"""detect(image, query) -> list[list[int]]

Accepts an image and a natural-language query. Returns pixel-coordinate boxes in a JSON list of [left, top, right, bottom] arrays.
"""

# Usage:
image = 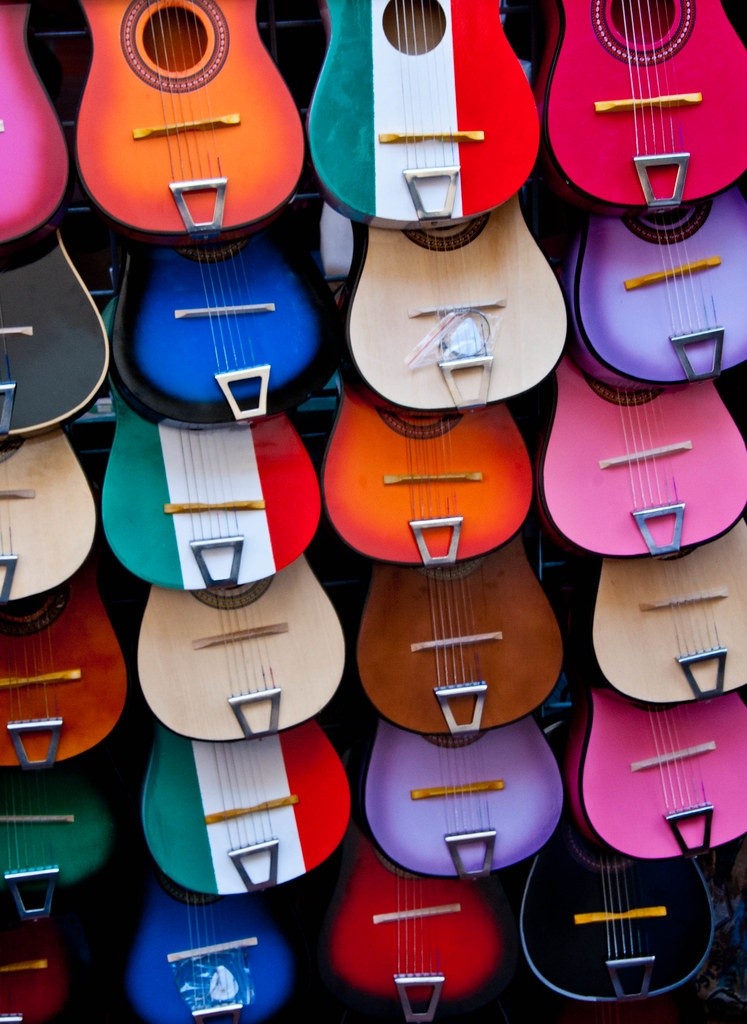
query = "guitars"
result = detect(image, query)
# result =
[[0, 2, 746, 1017]]
